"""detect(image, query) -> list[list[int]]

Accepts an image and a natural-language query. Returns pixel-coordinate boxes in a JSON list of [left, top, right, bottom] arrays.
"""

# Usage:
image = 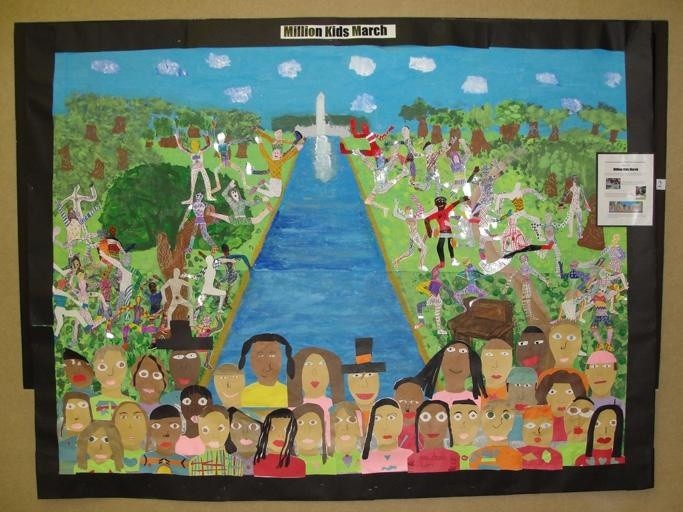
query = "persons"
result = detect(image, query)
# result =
[[51, 113, 627, 478]]
